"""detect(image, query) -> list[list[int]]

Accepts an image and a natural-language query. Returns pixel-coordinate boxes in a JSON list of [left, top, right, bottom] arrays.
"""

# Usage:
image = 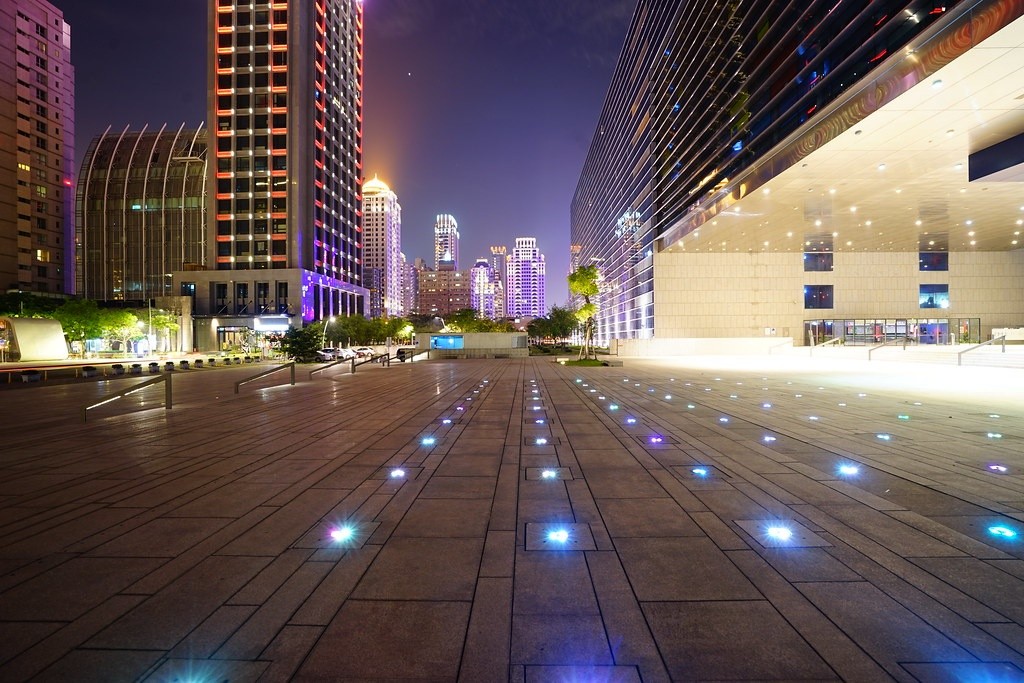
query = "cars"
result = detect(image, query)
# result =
[[294, 350, 332, 363], [354, 347, 375, 357], [322, 347, 365, 359]]
